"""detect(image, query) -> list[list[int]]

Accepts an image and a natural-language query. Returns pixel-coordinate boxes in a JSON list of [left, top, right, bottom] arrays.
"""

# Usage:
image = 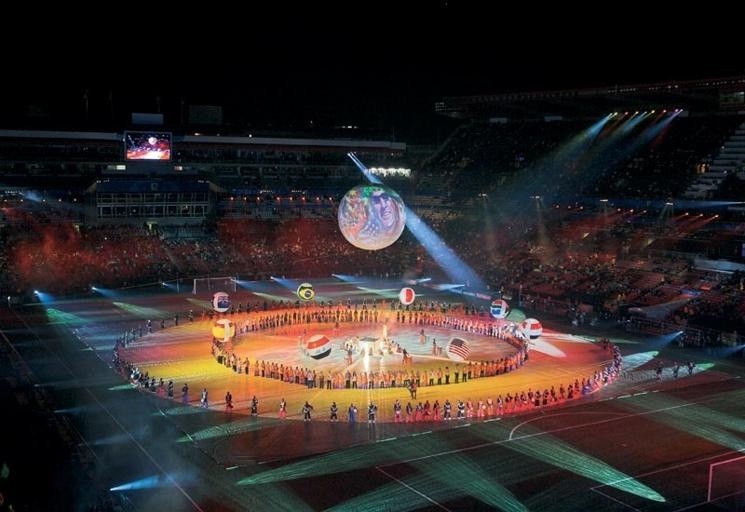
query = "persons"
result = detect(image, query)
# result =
[[409, 119, 744, 350], [671, 361, 681, 380], [1, 135, 408, 291], [653, 359, 666, 385], [686, 358, 694, 376], [110, 298, 621, 426]]
